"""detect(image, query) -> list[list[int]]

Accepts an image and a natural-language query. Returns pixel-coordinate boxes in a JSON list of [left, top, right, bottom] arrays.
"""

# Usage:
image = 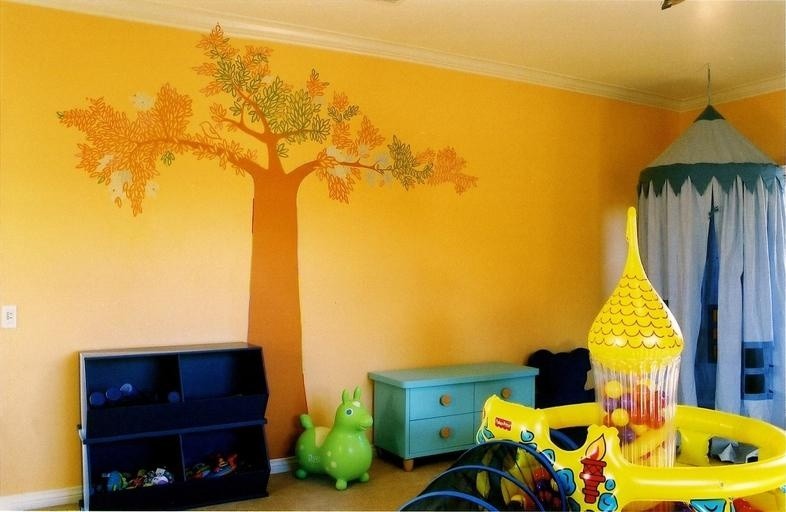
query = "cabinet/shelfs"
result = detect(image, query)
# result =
[[367, 361, 540, 472], [75, 342, 270, 510]]
